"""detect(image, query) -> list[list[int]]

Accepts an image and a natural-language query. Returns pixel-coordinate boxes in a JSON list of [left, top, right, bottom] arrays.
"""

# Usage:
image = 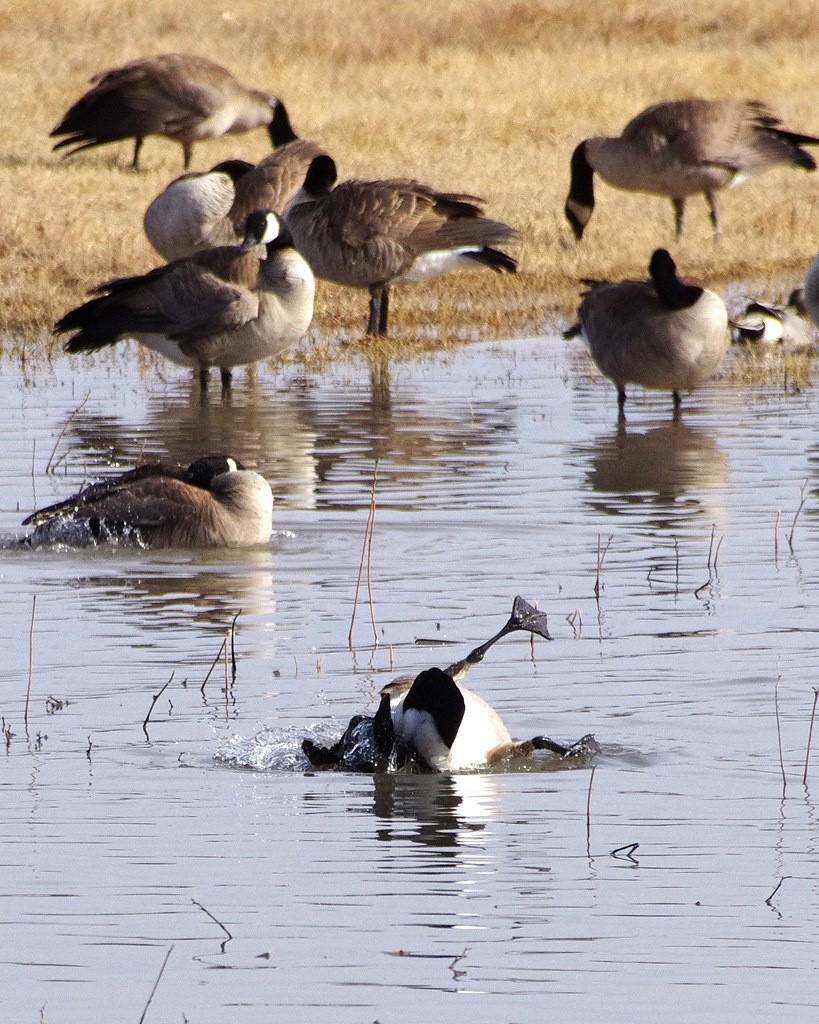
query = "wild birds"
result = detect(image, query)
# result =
[[52, 208, 316, 392], [282, 156, 521, 338], [144, 139, 326, 265], [47, 54, 302, 175], [17, 455, 273, 552], [726, 288, 816, 349], [561, 247, 732, 412], [563, 98, 819, 245], [302, 593, 604, 773]]
